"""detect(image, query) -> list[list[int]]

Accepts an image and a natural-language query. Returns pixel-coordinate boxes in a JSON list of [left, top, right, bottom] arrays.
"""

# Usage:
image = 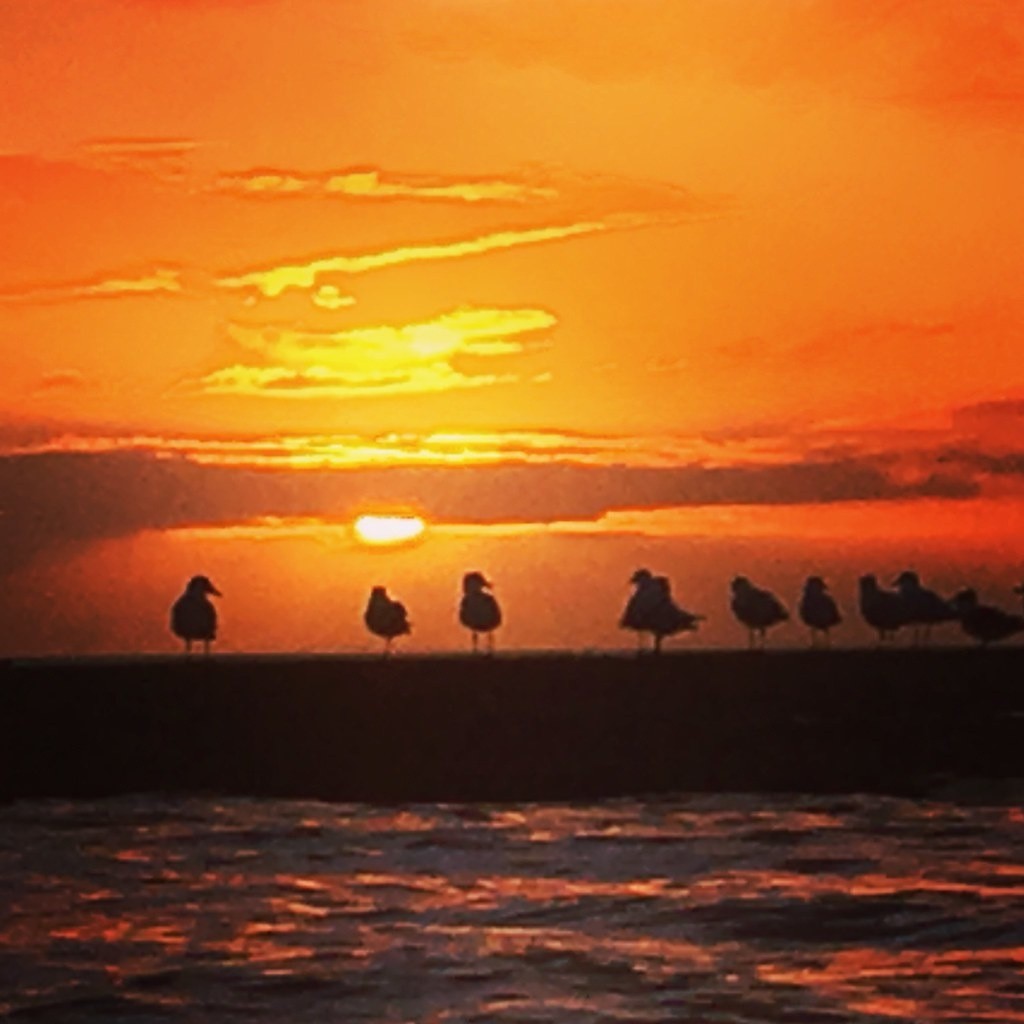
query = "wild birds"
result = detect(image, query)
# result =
[[362, 584, 412, 660], [857, 570, 1024, 650], [621, 568, 707, 661], [169, 574, 224, 664], [729, 574, 790, 657], [458, 570, 502, 662], [799, 575, 843, 653]]
